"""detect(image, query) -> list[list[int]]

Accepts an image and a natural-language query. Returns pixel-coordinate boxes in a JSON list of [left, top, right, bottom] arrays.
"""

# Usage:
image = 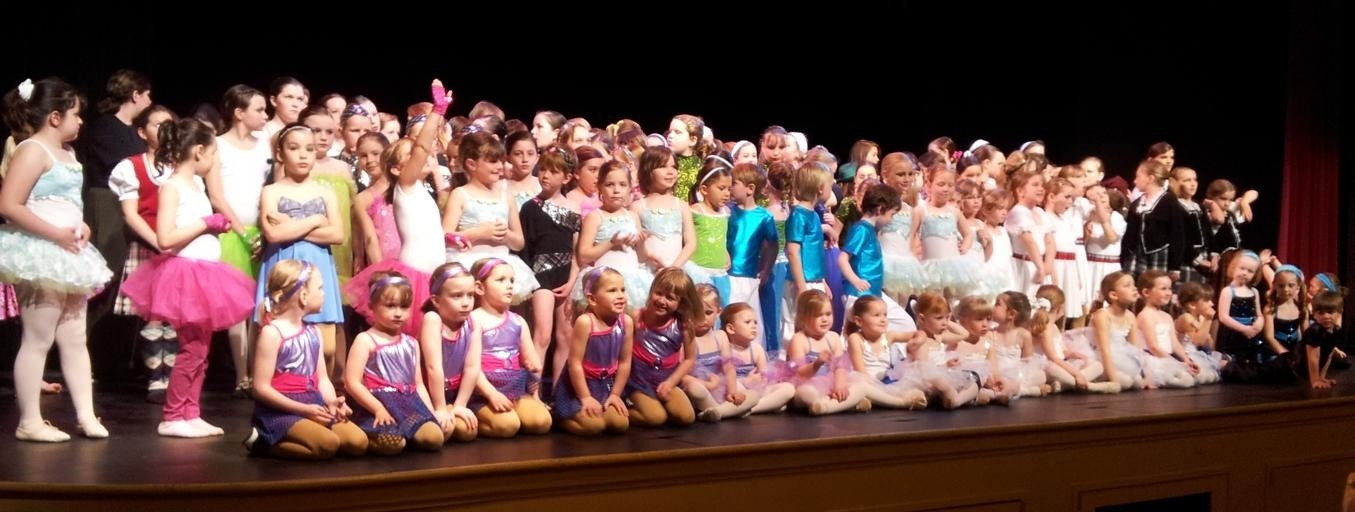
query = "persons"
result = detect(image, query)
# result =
[[0, 63, 1354, 444]]
